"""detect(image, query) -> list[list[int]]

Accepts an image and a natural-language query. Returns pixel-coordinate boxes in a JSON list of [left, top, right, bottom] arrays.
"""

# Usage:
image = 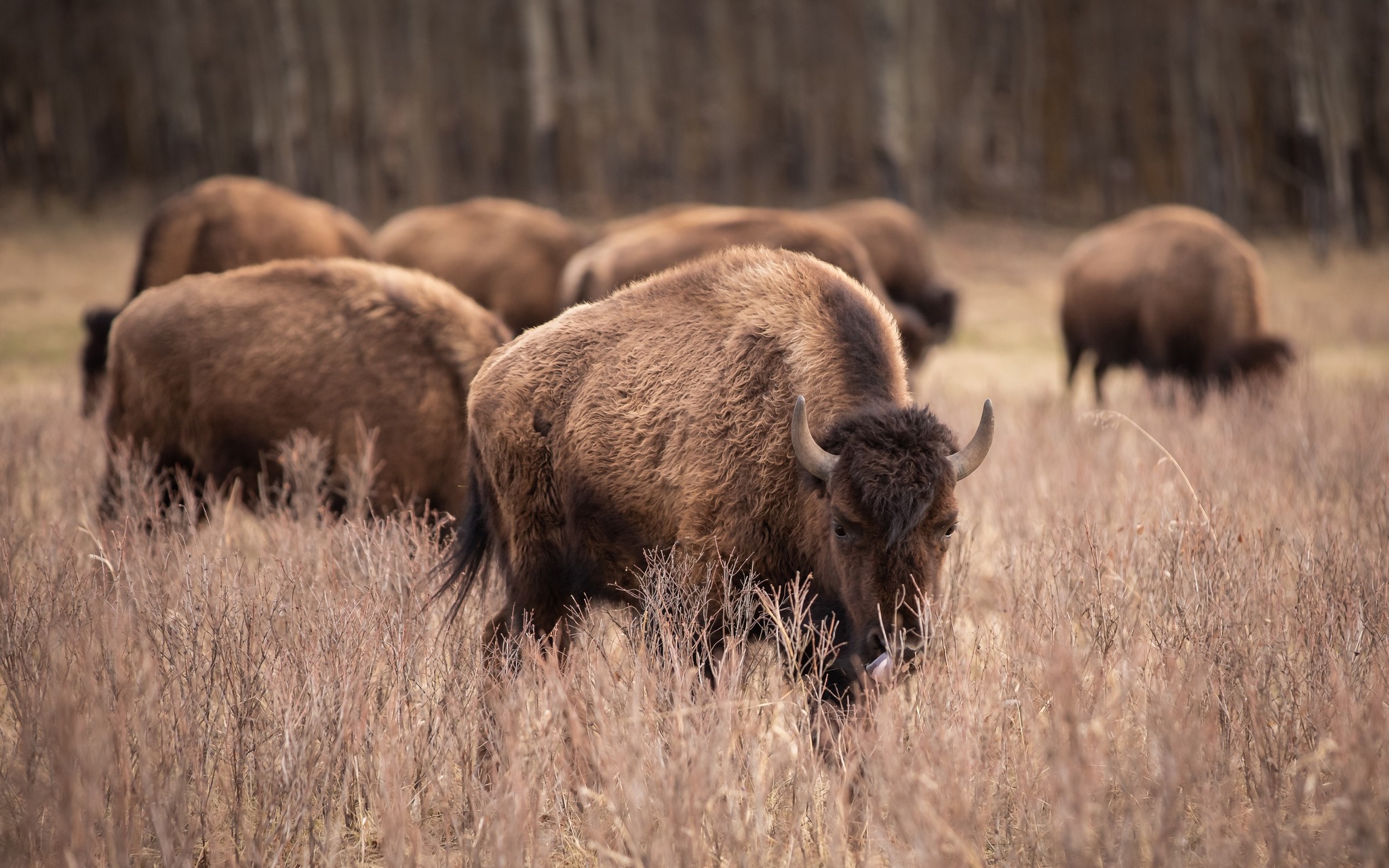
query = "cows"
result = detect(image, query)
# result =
[[409, 241, 992, 693], [81, 172, 957, 418], [102, 256, 515, 525], [1060, 200, 1294, 412]]
